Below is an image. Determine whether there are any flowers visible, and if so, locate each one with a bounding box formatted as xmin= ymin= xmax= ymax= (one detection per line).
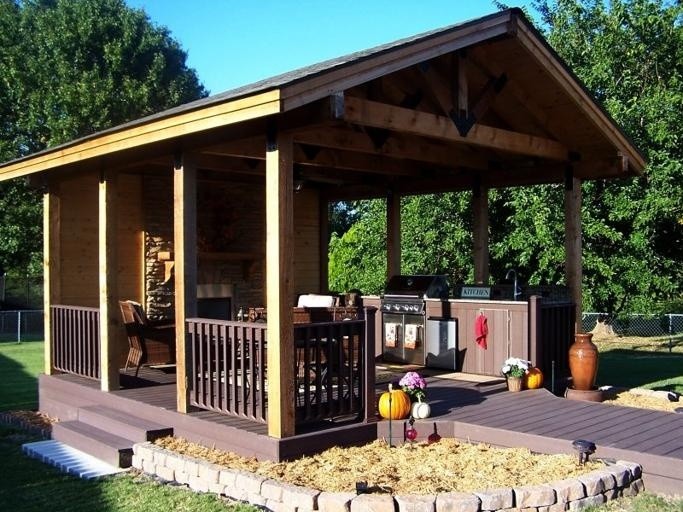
xmin=500 ymin=355 xmax=532 ymax=377
xmin=398 ymin=371 xmax=427 ymax=400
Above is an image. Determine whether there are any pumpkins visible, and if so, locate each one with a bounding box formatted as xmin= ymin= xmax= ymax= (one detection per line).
xmin=411 ymin=394 xmax=431 ymax=419
xmin=378 ymin=383 xmax=411 ymax=420
xmin=524 ymin=364 xmax=543 ymax=389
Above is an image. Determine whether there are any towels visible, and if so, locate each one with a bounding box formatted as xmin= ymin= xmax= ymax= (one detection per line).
xmin=384 ymin=322 xmax=418 ymax=350
xmin=473 ymin=313 xmax=489 ymax=351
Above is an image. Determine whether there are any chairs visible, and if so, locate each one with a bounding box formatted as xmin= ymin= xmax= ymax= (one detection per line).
xmin=116 ymin=298 xmax=211 ymax=379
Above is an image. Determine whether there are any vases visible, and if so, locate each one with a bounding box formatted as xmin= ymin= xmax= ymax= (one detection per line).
xmin=505 ymin=374 xmax=522 ymax=393
xmin=568 ymin=333 xmax=599 ymax=390
